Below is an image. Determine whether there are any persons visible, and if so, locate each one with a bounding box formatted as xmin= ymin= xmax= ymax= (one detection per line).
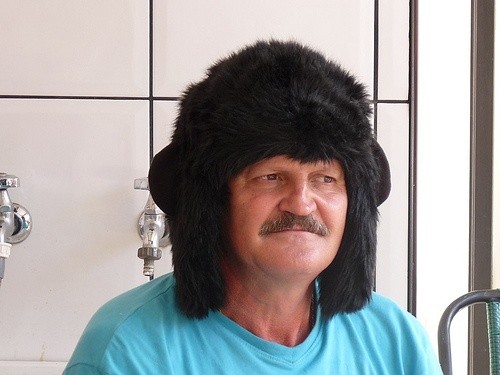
xmin=61 ymin=38 xmax=446 ymax=375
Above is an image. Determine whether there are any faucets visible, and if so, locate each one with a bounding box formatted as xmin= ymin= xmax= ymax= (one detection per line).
xmin=134 ymin=178 xmax=170 ymax=282
xmin=0 ymin=172 xmax=32 ymax=285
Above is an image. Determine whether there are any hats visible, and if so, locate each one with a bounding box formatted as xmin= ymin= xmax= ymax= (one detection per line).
xmin=147 ymin=37 xmax=391 ymax=321
